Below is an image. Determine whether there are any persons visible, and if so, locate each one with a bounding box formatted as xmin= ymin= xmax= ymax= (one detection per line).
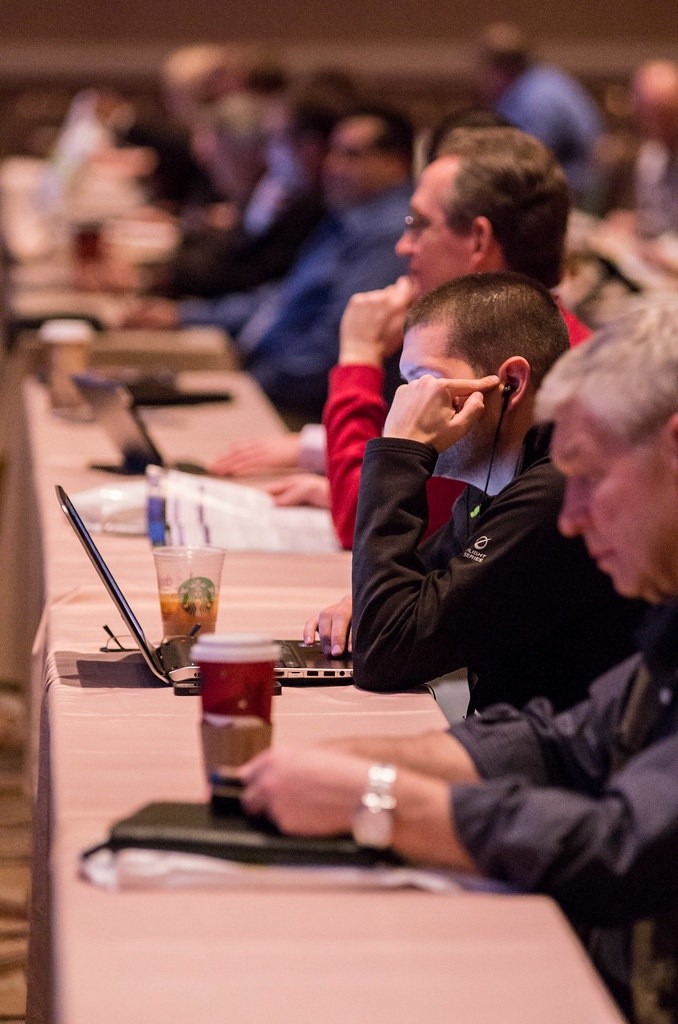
xmin=99 ymin=20 xmax=678 ymax=1024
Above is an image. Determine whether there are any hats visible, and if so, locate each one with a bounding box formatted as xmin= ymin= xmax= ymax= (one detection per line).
xmin=496 ymin=69 xmax=608 ymax=189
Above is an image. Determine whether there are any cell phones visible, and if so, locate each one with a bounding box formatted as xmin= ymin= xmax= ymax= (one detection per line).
xmin=209 ymin=774 xmax=245 ymax=797
xmin=174 ymin=682 xmax=282 ymax=696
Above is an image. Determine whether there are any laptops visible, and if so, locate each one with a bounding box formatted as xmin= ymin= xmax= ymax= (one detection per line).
xmin=107 ymin=801 xmax=406 ymax=870
xmin=55 ymin=483 xmax=358 ymax=683
xmin=70 ymin=375 xmax=221 ymax=479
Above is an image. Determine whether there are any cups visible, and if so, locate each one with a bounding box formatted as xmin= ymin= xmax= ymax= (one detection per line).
xmin=153 ymin=545 xmax=226 ymax=637
xmin=190 ymin=632 xmax=283 ymax=798
xmin=36 ymin=318 xmax=95 ymax=418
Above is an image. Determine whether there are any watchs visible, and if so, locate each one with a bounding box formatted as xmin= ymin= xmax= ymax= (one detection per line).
xmin=350 ymin=762 xmax=399 ymax=849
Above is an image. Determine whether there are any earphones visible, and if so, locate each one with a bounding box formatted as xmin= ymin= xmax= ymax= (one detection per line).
xmin=501 ymin=385 xmax=517 ymax=409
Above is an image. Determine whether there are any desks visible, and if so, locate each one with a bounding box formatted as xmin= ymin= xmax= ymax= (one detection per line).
xmin=0 ymin=370 xmax=631 ymax=1024
xmin=0 ymin=152 xmax=241 ymax=371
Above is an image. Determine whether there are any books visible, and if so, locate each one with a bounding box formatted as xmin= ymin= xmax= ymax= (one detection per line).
xmin=146 ymin=464 xmax=343 ymax=553
xmin=109 ymin=802 xmax=377 ymax=865
